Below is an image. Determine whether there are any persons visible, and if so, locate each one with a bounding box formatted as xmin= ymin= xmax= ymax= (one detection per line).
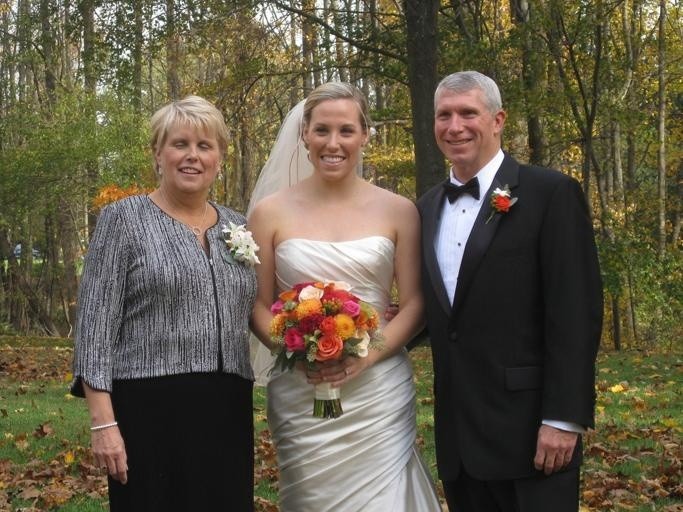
xmin=246 ymin=83 xmax=440 ymax=512
xmin=70 ymin=95 xmax=399 ymax=512
xmin=402 ymin=70 xmax=603 ymax=512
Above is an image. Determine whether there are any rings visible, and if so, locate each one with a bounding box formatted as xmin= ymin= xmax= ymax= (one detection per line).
xmin=100 ymin=465 xmax=106 ymax=470
xmin=344 ymin=369 xmax=348 ymax=375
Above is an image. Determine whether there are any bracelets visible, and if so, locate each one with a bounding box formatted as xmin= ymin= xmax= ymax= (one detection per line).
xmin=88 ymin=421 xmax=120 ymax=431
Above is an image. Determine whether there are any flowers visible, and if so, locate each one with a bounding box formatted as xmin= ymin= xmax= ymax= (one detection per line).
xmin=269 ymin=282 xmax=386 ymax=419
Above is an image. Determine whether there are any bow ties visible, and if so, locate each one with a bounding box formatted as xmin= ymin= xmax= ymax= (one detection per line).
xmin=443 ymin=177 xmax=482 ymax=205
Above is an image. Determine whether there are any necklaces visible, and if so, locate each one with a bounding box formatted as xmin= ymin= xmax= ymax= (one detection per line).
xmin=159 ymin=192 xmax=207 ymax=237
